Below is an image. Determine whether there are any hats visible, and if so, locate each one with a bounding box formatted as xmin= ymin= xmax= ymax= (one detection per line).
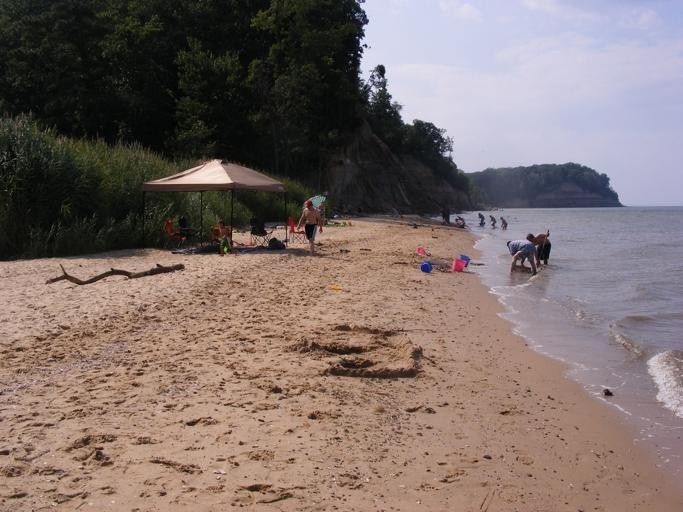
xmin=305 ymin=200 xmax=313 ymax=207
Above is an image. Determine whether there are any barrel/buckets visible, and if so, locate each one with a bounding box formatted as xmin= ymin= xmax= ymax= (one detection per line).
xmin=453 ymin=257 xmax=466 ymax=271
xmin=421 ymin=261 xmax=432 ymax=272
xmin=461 ymin=254 xmax=472 ymax=267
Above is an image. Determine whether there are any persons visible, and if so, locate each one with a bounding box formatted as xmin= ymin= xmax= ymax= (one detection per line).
xmin=525 ymin=229 xmax=550 ymax=266
xmin=438 ymin=207 xmax=468 ymax=229
xmin=489 ymin=215 xmax=496 ymax=229
xmin=500 ymin=217 xmax=506 ymax=230
xmin=296 ymin=200 xmax=323 ymax=256
xmin=287 ymin=213 xmax=294 ymax=234
xmin=506 ymin=240 xmax=537 ymax=276
xmin=212 ymin=221 xmax=229 ymax=251
xmin=163 ymin=215 xmax=186 ymax=242
xmin=477 ymin=213 xmax=485 ymax=226
xmin=316 ymin=202 xmax=324 ymax=227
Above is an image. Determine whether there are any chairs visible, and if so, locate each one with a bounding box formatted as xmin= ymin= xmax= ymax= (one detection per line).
xmin=288 ymin=216 xmax=307 ymax=244
xmin=177 ymin=212 xmax=204 ymax=251
xmin=250 ymin=217 xmax=272 ymax=247
